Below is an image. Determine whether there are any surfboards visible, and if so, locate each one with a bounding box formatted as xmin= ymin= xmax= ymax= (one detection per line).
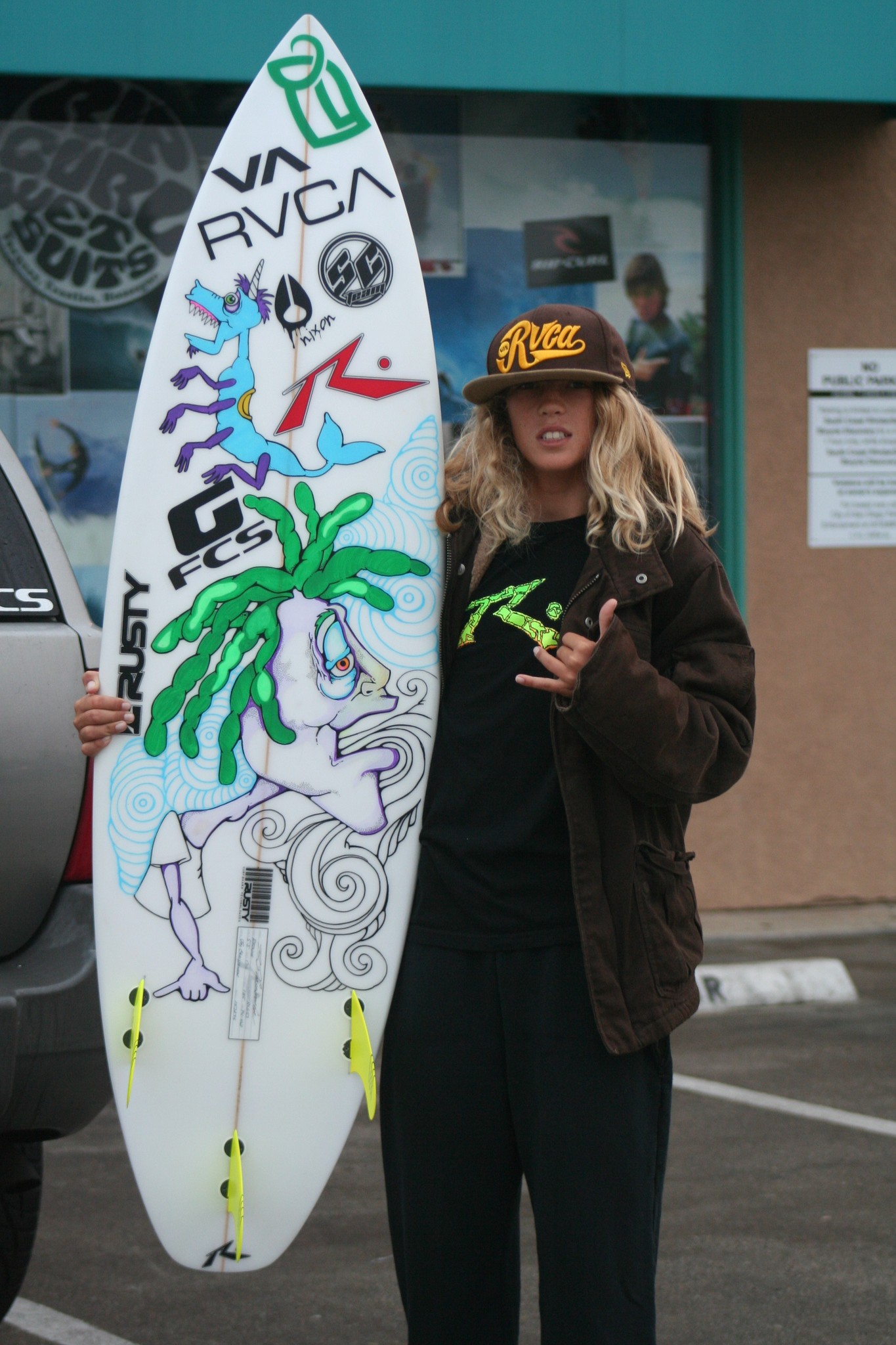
xmin=92 ymin=14 xmax=444 ymax=1275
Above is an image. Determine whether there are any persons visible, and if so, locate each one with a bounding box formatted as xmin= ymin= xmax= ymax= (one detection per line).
xmin=621 ymin=250 xmax=693 ymax=415
xmin=41 ymin=419 xmax=87 ymax=498
xmin=75 ymin=304 xmax=755 ymax=1345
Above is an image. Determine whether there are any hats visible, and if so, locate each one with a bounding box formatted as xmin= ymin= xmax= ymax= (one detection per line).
xmin=461 ymin=304 xmax=639 ymax=406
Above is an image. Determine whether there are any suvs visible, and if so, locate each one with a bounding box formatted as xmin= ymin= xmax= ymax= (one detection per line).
xmin=1 ymin=427 xmax=115 ymax=1320
